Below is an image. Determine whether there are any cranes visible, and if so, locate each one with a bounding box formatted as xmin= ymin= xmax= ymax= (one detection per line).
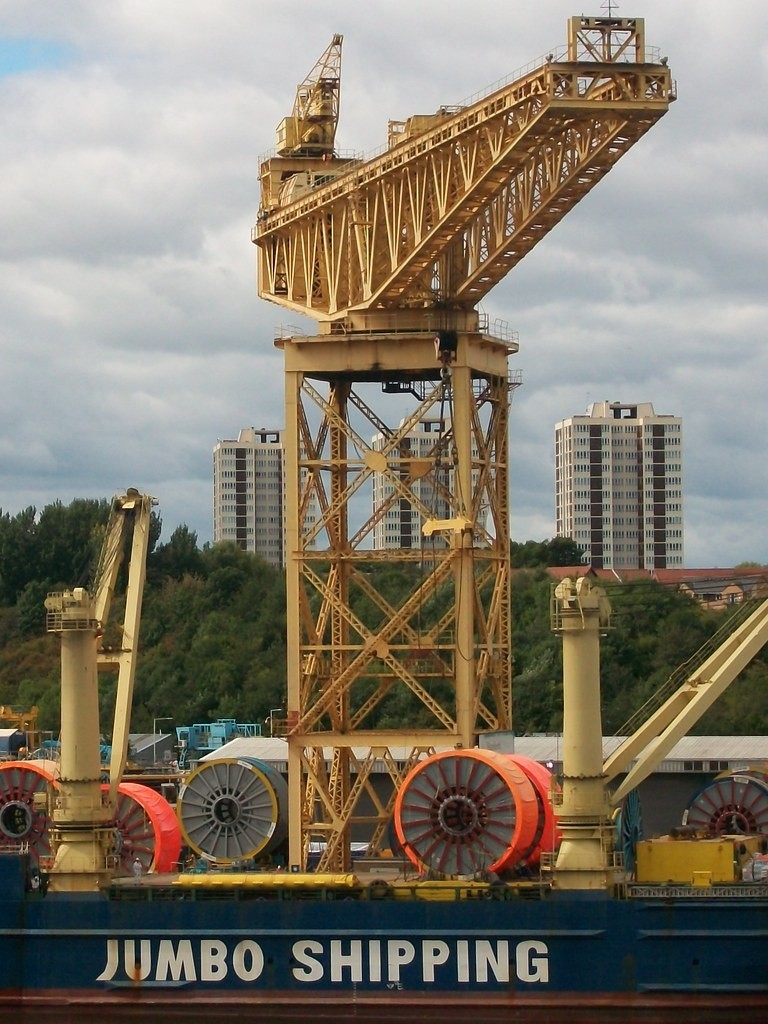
xmin=249 ymin=1 xmax=677 ymax=900
